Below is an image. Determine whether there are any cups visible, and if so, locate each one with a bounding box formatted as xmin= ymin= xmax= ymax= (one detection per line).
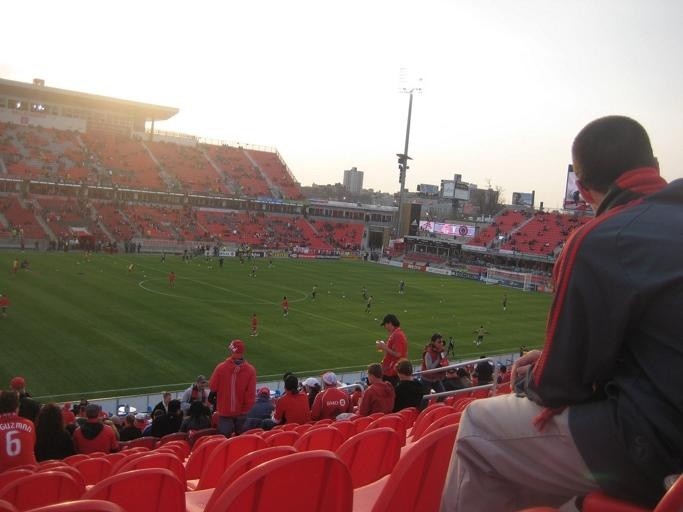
xmin=440 ymin=352 xmax=445 ymax=359
xmin=375 ymin=341 xmax=383 ymax=352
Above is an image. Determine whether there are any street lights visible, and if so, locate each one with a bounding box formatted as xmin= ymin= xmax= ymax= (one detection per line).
xmin=392 ymin=81 xmax=422 ymax=246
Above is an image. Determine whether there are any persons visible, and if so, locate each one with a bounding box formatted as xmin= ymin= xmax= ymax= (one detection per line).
xmin=3 ymin=117 xmax=682 ymax=509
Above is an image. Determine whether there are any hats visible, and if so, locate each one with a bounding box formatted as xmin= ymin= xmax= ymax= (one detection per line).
xmin=302 ymin=373 xmax=337 ymax=388
xmin=134 ymin=413 xmax=144 ymax=419
xmin=230 ymin=339 xmax=244 ymax=354
xmin=197 ymin=376 xmax=208 ymax=383
xmin=11 ymin=377 xmax=24 ymax=388
xmin=258 ymin=388 xmax=270 ymax=395
xmin=380 ymin=315 xmax=396 ymax=326
